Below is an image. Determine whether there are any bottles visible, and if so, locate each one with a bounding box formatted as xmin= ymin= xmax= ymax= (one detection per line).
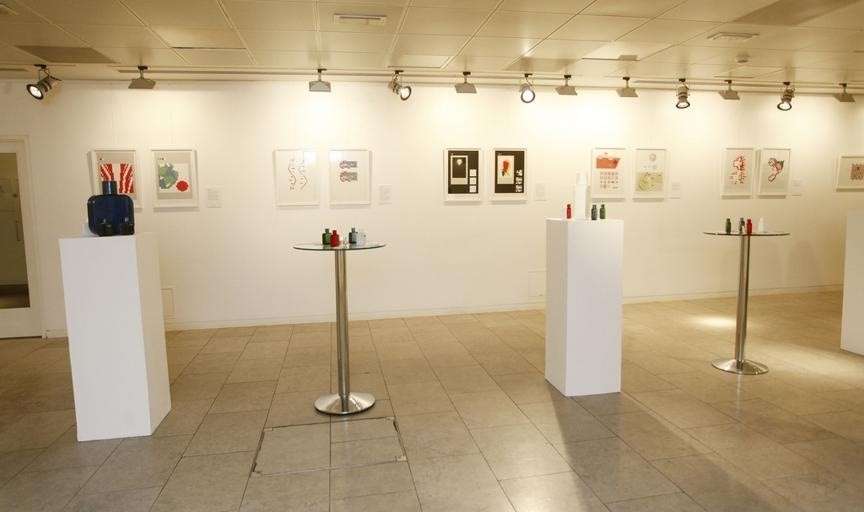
xmin=565 ymin=199 xmax=609 ymax=222
xmin=319 ymin=224 xmax=368 ymax=246
xmin=715 ymin=211 xmax=770 ymax=236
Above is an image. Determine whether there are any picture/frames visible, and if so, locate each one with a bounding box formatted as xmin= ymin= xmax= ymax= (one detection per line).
xmin=327 ymin=148 xmax=371 ymax=207
xmin=89 ymin=148 xmax=144 ymax=210
xmin=150 ymin=147 xmax=199 ymax=209
xmin=443 ymin=147 xmax=483 ymax=203
xmin=589 ymin=145 xmax=627 ymax=199
xmin=720 ymin=147 xmax=756 ymax=196
xmin=836 ymin=155 xmax=864 ymax=190
xmin=273 ymin=148 xmax=320 ymax=206
xmin=757 ymin=147 xmax=792 ymax=196
xmin=488 ymin=148 xmax=527 ymax=202
xmin=631 ymin=146 xmax=667 ymax=199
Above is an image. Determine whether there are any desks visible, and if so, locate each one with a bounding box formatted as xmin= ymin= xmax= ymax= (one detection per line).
xmin=293 ymin=242 xmax=387 ymax=417
xmin=704 ymin=231 xmax=790 ymax=375
